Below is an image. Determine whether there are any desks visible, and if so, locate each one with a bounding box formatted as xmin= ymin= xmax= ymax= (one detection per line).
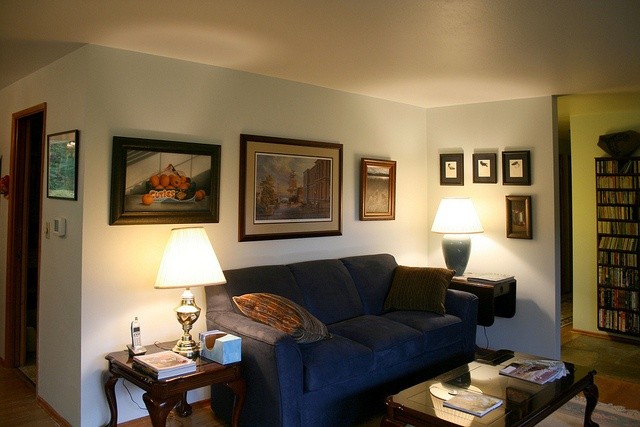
xmin=104 ymin=340 xmax=246 ymax=427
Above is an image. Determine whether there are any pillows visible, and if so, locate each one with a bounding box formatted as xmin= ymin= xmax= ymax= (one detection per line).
xmin=232 ymin=292 xmax=334 ymax=345
xmin=382 ymin=265 xmax=457 ymax=317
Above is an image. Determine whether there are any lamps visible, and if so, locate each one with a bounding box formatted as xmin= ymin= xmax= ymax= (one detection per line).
xmin=431 ymin=198 xmax=485 ymax=276
xmin=153 ymin=227 xmax=228 ymax=359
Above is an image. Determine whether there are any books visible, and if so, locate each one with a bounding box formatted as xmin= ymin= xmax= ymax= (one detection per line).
xmin=442 ymin=391 xmax=504 ymax=416
xmin=596 ymin=160 xmax=639 ymax=174
xmin=598 ymin=250 xmax=638 ymax=268
xmin=597 ymin=191 xmax=639 ymax=205
xmin=598 ymin=287 xmax=639 ymax=311
xmin=467 ymin=272 xmax=515 ymax=285
xmin=497 ymin=357 xmax=569 ymax=386
xmin=596 ymin=176 xmax=640 ymax=190
xmin=132 ymin=350 xmax=199 ymax=380
xmin=598 ymin=207 xmax=633 ymax=220
xmin=598 ymin=222 xmax=638 ymax=236
xmin=598 ymin=266 xmax=639 ymax=290
xmin=598 ymin=237 xmax=638 ymax=252
xmin=599 ymin=308 xmax=640 ymax=338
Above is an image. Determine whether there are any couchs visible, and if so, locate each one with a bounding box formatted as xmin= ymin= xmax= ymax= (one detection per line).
xmin=205 ymin=253 xmax=479 ymax=427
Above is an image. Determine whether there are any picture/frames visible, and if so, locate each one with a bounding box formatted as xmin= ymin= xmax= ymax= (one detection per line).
xmin=238 ymin=133 xmax=343 ymax=242
xmin=47 ymin=129 xmax=80 ymax=201
xmin=472 ymin=153 xmax=497 ymax=183
xmin=359 ymin=157 xmax=398 ymax=221
xmin=502 ymin=150 xmax=531 ymax=186
xmin=440 ymin=153 xmax=464 ymax=186
xmin=109 ymin=135 xmax=222 ymax=226
xmin=505 ymin=195 xmax=533 ymax=240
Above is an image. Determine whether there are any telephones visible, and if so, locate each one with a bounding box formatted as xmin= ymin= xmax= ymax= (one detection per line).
xmin=126 ymin=317 xmax=148 ymax=358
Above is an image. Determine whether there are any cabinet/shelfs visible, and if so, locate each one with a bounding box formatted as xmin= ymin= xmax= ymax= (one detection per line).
xmin=595 ymin=158 xmax=640 ymax=339
